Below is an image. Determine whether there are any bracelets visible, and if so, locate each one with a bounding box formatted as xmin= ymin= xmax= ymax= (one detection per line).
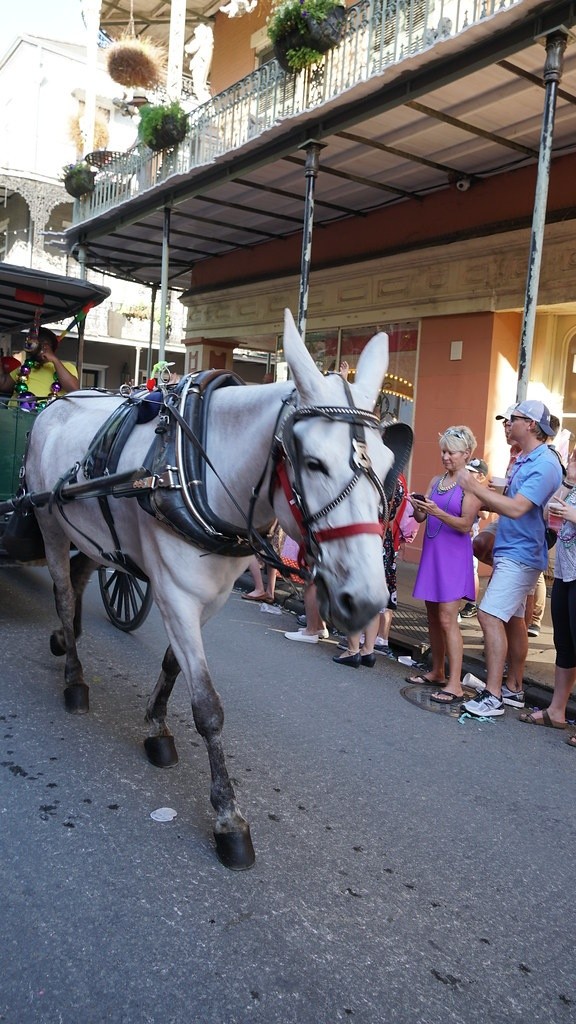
xmin=562 ymin=480 xmax=573 ymax=489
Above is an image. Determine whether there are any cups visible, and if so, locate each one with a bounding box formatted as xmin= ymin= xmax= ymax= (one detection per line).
xmin=463 ymin=673 xmax=486 ymax=691
xmin=547 ymin=502 xmax=563 ymax=530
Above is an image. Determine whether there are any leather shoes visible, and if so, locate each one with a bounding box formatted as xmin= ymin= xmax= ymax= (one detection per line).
xmin=360 ymin=652 xmax=376 ymax=667
xmin=333 ymin=653 xmax=361 ymax=670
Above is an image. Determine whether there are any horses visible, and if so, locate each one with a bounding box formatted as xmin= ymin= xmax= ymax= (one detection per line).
xmin=25 ymin=308 xmax=393 ymax=871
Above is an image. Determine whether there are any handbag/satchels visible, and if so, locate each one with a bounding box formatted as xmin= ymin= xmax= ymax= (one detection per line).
xmin=545 ymin=529 xmax=558 ymax=550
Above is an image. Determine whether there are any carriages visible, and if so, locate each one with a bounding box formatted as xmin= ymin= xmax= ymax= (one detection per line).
xmin=0 ymin=263 xmax=390 ymax=870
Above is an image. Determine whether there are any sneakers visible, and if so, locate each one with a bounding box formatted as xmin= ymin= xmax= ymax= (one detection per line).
xmin=527 ymin=625 xmax=540 ymax=636
xmin=476 ymin=683 xmax=526 ymax=708
xmin=460 ymin=603 xmax=477 ymax=618
xmin=461 ymin=690 xmax=505 ymax=717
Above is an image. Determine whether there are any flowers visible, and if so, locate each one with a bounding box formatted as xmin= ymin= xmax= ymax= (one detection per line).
xmin=268 ymin=0 xmax=348 ymax=47
xmin=57 ymin=163 xmax=91 ymax=186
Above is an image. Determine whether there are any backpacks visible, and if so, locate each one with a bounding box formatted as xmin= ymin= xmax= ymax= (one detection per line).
xmin=390 ymin=475 xmax=420 ymax=551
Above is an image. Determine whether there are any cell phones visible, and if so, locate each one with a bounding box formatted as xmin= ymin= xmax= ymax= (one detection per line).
xmin=411 ymin=494 xmax=427 ymax=504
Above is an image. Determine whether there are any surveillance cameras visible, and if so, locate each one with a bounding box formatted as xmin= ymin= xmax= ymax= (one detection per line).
xmin=457 ymin=178 xmax=470 ymax=192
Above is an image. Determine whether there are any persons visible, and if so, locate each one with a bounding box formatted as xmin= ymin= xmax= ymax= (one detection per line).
xmin=185 ymin=24 xmax=214 ymax=104
xmin=241 ymin=556 xmax=277 ymax=604
xmin=128 ymin=90 xmax=153 ymax=191
xmin=284 ymin=473 xmax=408 ymax=669
xmin=520 ymin=445 xmax=576 ymax=748
xmin=404 ymin=425 xmax=482 ymax=703
xmin=0 ymin=327 xmax=79 ymax=410
xmin=458 ymin=399 xmax=564 ymax=717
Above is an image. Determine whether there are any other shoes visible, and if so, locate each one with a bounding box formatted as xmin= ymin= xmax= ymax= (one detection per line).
xmin=240 ymin=591 xmax=266 ymax=602
xmin=338 ymin=638 xmax=390 ymax=655
xmin=297 ymin=615 xmax=307 ymax=625
xmin=284 ymin=631 xmax=319 ymax=643
xmin=263 ymin=596 xmax=275 ymax=604
xmin=298 ymin=627 xmax=330 ymax=639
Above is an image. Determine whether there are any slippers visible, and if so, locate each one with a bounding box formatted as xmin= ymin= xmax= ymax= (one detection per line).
xmin=431 ymin=689 xmax=464 ymax=703
xmin=519 ymin=708 xmax=567 ymax=729
xmin=567 ymin=734 xmax=576 ymax=746
xmin=405 ymin=674 xmax=446 ymax=687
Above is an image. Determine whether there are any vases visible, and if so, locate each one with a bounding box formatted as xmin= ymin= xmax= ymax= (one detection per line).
xmin=64 ymin=171 xmax=95 ymax=198
xmin=271 ymin=6 xmax=344 ymax=73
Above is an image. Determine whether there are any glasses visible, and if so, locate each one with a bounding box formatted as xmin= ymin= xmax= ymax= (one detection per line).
xmin=502 ymin=422 xmax=506 ymax=427
xmin=510 ymin=414 xmax=531 ymax=422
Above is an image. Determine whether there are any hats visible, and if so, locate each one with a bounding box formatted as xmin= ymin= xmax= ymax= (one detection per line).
xmin=495 ymin=403 xmax=518 ymax=421
xmin=515 ymin=400 xmax=556 ymax=435
xmin=464 ymin=459 xmax=488 ymax=476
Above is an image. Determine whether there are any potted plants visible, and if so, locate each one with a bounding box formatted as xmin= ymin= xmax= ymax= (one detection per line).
xmin=137 ymin=99 xmax=189 ymax=152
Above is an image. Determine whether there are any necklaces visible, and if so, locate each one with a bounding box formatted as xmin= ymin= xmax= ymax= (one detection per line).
xmin=16 ymin=361 xmax=62 ymax=408
xmin=426 ymin=472 xmax=457 ymax=538
